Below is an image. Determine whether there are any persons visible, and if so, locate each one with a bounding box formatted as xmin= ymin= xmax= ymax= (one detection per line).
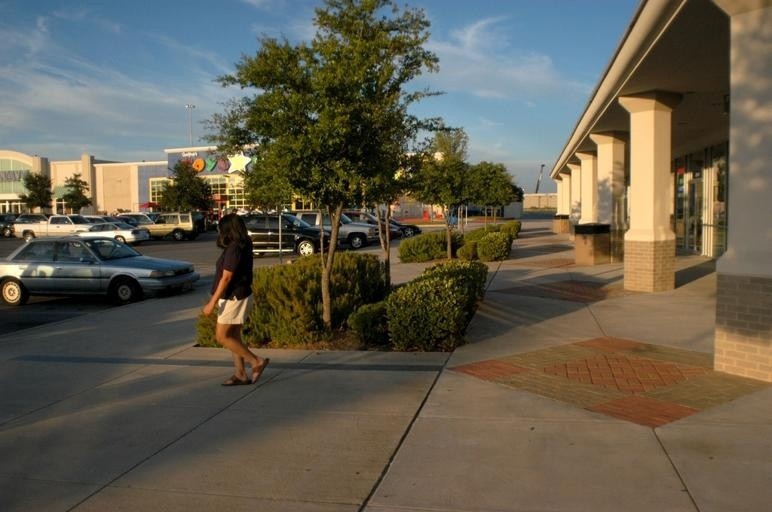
xmin=202 ymin=213 xmax=269 ymax=386
xmin=232 ymin=208 xmax=238 ymax=214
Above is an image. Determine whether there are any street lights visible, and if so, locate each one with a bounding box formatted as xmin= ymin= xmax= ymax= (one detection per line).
xmin=184 ymin=104 xmax=196 ymax=146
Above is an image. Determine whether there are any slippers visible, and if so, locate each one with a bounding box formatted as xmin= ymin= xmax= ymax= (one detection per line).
xmin=221 ymin=374 xmax=251 ymax=386
xmin=252 ymin=358 xmax=269 ymax=383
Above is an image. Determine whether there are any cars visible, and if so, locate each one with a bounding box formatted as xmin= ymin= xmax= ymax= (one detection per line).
xmin=241 ymin=210 xmax=422 ymax=256
xmin=0 ymin=211 xmax=207 ymax=305
